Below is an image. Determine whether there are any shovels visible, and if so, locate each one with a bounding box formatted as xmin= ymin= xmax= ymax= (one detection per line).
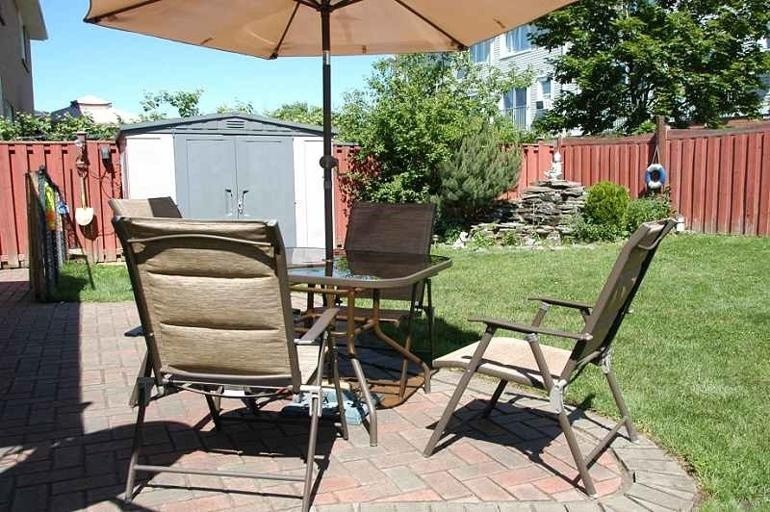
xmin=75 ymin=167 xmax=94 ymax=227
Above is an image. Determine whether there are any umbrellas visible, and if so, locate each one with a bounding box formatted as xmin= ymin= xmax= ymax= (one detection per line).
xmin=82 ymin=0 xmax=576 ymax=426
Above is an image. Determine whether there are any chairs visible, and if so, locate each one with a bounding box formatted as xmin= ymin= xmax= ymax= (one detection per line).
xmin=422 ymin=218 xmax=676 ymax=500
xmin=312 ymin=204 xmax=436 ymax=368
xmin=108 ymin=196 xmax=182 ymax=218
xmin=111 ymin=217 xmax=348 ymax=512
